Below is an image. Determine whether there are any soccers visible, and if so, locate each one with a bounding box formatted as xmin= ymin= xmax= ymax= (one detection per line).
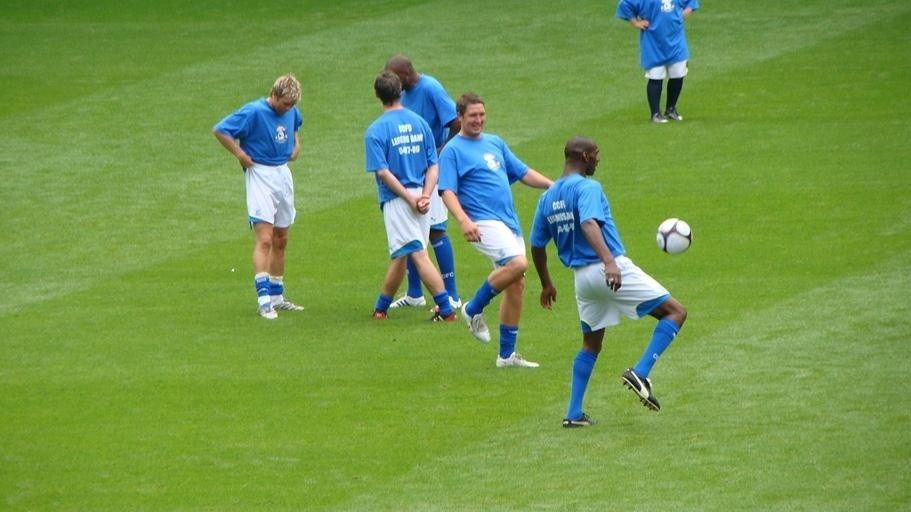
xmin=656 ymin=218 xmax=691 ymax=254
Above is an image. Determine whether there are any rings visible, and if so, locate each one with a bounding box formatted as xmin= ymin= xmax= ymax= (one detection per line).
xmin=608 ymin=278 xmax=614 ymax=283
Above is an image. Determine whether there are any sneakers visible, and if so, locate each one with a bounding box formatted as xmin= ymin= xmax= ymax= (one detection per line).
xmin=259 ymin=302 xmax=278 ymax=319
xmin=651 ymin=112 xmax=668 ymax=123
xmin=373 ymin=310 xmax=389 ymax=320
xmin=496 ymin=352 xmax=539 ymax=368
xmin=273 ymin=300 xmax=304 ymax=310
xmin=665 ymin=107 xmax=683 ymax=120
xmin=563 ymin=413 xmax=597 ymax=428
xmin=623 ymin=367 xmax=660 ymax=412
xmin=390 ymin=293 xmax=426 ymax=308
xmin=431 ymin=296 xmax=461 ymax=322
xmin=461 ymin=301 xmax=491 ymax=343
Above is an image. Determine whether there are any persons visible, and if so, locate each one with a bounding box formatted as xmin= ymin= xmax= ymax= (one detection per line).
xmin=614 ymin=0 xmax=700 ymax=123
xmin=383 ymin=52 xmax=462 ymax=311
xmin=212 ymin=71 xmax=306 ymax=320
xmin=529 ymin=132 xmax=686 ymax=429
xmin=436 ymin=89 xmax=556 ymax=367
xmin=364 ymin=68 xmax=458 ymax=322
